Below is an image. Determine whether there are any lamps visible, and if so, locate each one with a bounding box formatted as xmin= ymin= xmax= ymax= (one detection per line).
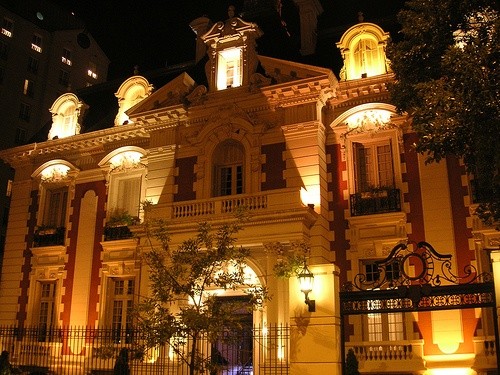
xmin=296 ymin=263 xmax=315 ymax=312
